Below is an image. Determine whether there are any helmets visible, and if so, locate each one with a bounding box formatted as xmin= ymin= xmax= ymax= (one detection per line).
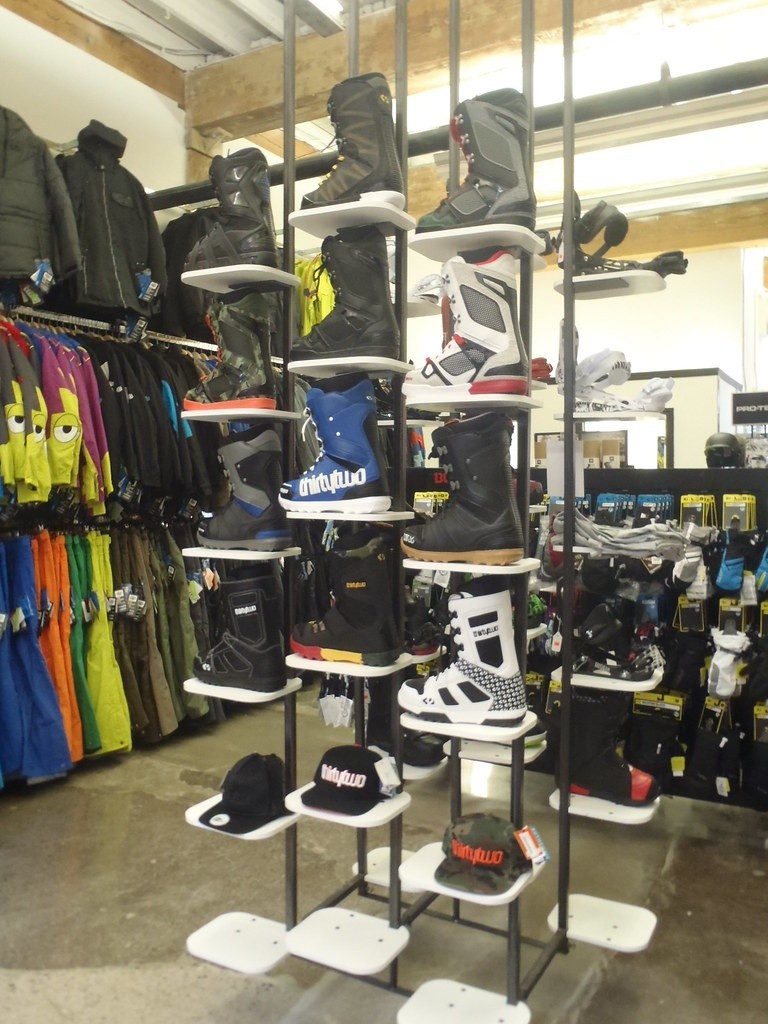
xmin=704 ymin=432 xmax=741 ymax=462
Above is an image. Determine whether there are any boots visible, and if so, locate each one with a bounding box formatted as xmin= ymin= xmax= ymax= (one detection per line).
xmin=299 ymin=72 xmax=405 ymax=211
xmin=288 ymin=226 xmax=400 ymax=359
xmin=398 ymin=575 xmax=528 ymax=725
xmin=181 ymin=147 xmax=277 ymax=272
xmin=416 ymin=88 xmax=536 ymax=233
xmin=276 ymin=371 xmax=393 ymax=514
xmin=180 ymin=286 xmax=276 ymax=410
xmin=288 ymin=526 xmax=401 ymax=666
xmin=367 ymin=663 xmax=446 ymax=766
xmin=553 ymin=684 xmax=661 ymax=805
xmin=399 ymin=246 xmax=528 ymax=395
xmin=399 ymin=410 xmax=525 ymax=565
xmin=197 ymin=424 xmax=293 ymax=550
xmin=194 ymin=558 xmax=287 ymax=692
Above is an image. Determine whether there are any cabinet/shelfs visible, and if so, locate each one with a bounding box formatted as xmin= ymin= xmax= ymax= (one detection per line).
xmin=181 ymin=0 xmax=669 ymax=1024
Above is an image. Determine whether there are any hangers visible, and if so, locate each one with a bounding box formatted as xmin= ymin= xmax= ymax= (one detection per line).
xmin=0 ymin=514 xmax=191 ymax=540
xmin=0 ymin=304 xmax=284 ymax=378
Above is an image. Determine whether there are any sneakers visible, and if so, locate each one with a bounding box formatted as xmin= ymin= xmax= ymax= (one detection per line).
xmin=402 ymin=594 xmax=440 ymax=655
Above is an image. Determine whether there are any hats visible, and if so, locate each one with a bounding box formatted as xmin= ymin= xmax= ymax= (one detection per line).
xmin=435 ymin=813 xmax=532 ymax=896
xmin=301 ymin=743 xmax=402 ymax=817
xmin=198 ymin=752 xmax=294 ymax=834
